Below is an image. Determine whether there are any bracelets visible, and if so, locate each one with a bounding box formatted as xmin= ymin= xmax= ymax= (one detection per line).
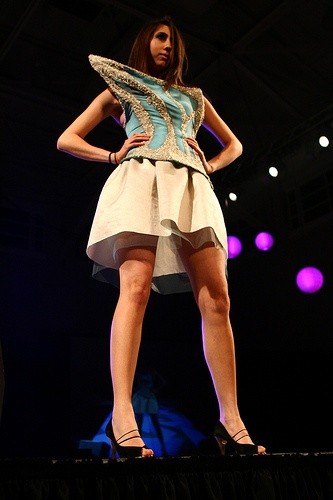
xmin=114 ymin=151 xmax=119 ymax=165
xmin=109 ymin=152 xmax=113 ymax=164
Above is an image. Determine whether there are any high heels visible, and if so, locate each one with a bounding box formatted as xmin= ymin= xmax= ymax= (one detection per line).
xmin=214 ymin=419 xmax=265 ymax=455
xmin=104 ymin=417 xmax=154 ymax=459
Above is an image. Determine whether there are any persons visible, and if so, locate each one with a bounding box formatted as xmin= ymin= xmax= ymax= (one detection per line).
xmin=56 ymin=14 xmax=271 ymax=456
